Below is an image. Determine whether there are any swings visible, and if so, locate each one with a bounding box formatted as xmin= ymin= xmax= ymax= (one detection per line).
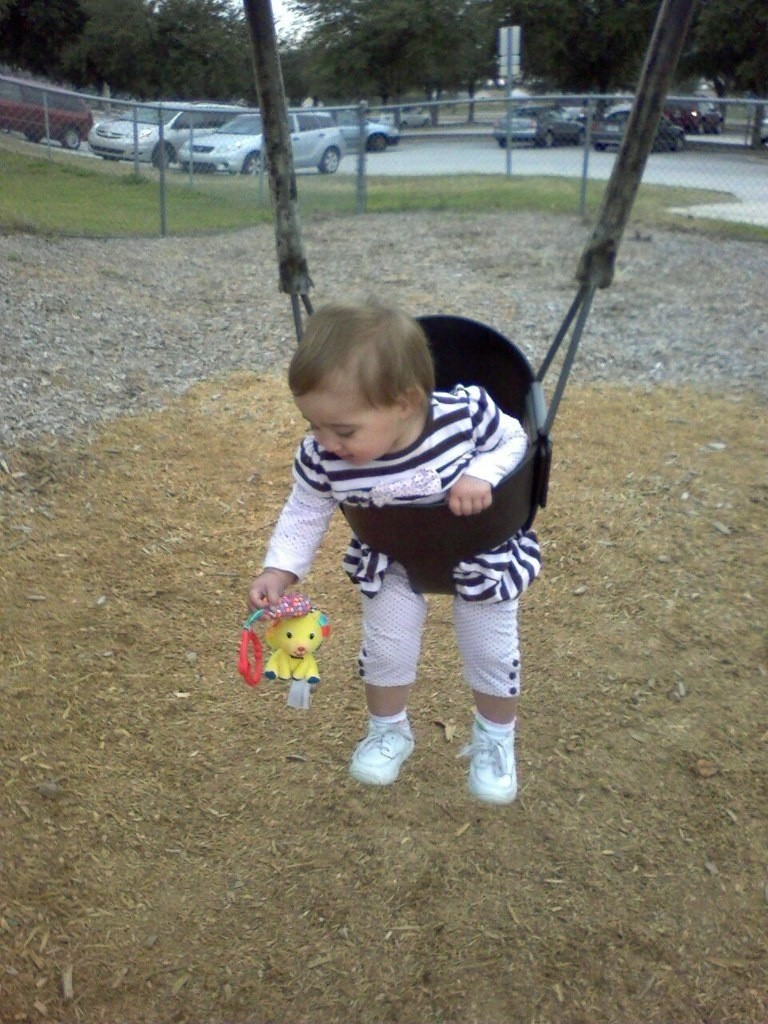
xmin=241 ymin=1 xmax=698 ymax=596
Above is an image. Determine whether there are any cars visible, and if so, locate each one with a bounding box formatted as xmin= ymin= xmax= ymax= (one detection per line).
xmin=493 ymin=105 xmax=587 ymax=149
xmin=662 ymin=95 xmax=725 ymax=135
xmin=586 ymin=102 xmax=687 ymax=154
xmin=380 ymin=106 xmax=431 ymax=128
xmin=176 ymin=109 xmax=344 ymax=177
xmin=333 ymin=108 xmax=402 ymax=155
xmin=0 ymin=79 xmax=94 ymax=150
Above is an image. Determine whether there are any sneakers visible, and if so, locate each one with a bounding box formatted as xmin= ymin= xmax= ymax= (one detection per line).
xmin=349 ymin=719 xmax=414 ymax=786
xmin=457 ymin=724 xmax=517 ymax=804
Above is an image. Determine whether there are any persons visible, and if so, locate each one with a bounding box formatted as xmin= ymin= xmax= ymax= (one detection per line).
xmin=248 ymin=303 xmax=542 ymax=806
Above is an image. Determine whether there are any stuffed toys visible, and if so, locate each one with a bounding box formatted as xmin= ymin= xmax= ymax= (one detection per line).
xmin=264 ymin=607 xmax=330 ymax=684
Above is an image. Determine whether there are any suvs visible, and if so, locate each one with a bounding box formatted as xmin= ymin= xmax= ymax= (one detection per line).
xmin=86 ymin=99 xmax=250 ymax=169
